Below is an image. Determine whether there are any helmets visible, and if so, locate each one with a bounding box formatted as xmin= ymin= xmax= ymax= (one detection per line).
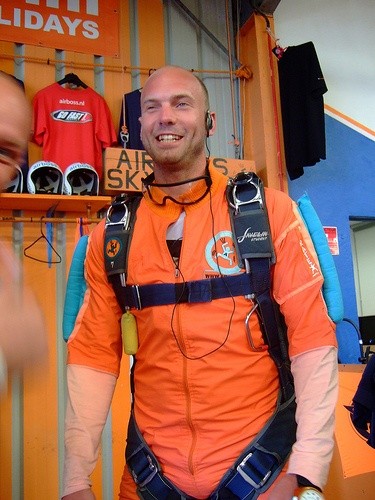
xmin=1 ymin=161 xmax=23 ymax=193
xmin=64 ymin=162 xmax=99 ymax=196
xmin=26 ymin=161 xmax=64 ymax=195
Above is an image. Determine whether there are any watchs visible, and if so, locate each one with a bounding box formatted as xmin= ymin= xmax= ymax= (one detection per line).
xmin=290 ymin=486 xmax=326 ymax=500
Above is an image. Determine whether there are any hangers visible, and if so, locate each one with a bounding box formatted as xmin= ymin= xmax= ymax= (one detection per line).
xmin=139 ymin=68 xmax=156 ymax=91
xmin=24 ymin=216 xmax=62 ymax=264
xmin=56 ymin=62 xmax=88 ymax=89
xmin=275 ymin=39 xmax=287 ymax=59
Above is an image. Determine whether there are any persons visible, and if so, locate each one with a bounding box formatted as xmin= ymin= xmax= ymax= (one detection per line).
xmin=0 ymin=69 xmax=48 ymax=377
xmin=65 ymin=67 xmax=338 ymax=500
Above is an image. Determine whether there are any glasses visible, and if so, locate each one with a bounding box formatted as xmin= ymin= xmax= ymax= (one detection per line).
xmin=141 ymin=157 xmax=212 ymax=207
